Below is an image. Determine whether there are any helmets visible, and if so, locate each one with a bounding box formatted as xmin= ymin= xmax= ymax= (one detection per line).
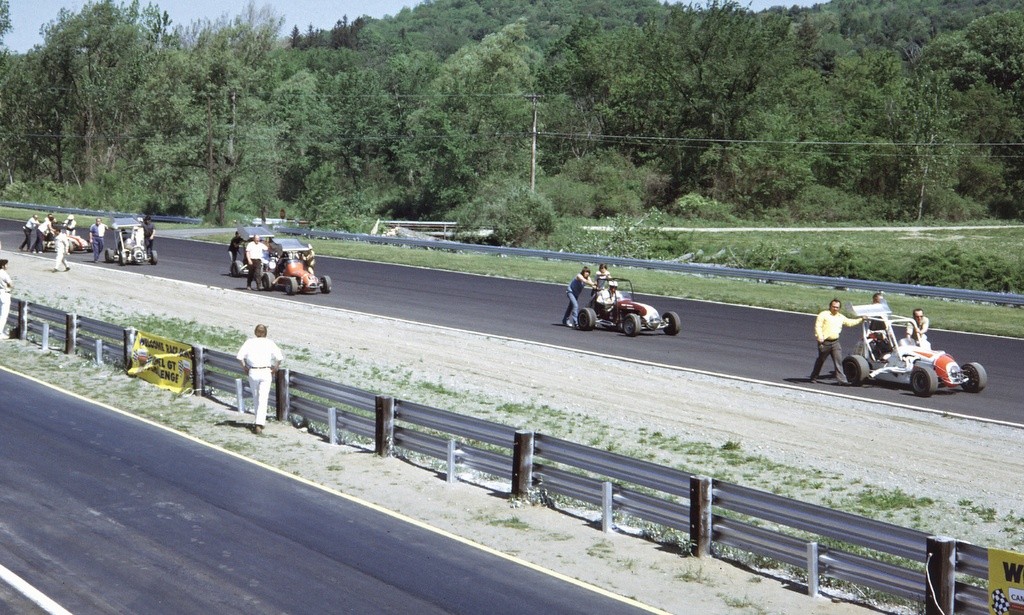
xmin=608 ymin=281 xmax=618 ymax=286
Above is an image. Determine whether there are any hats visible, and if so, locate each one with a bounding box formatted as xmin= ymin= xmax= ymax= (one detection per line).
xmin=68 ymin=214 xmax=74 ymax=220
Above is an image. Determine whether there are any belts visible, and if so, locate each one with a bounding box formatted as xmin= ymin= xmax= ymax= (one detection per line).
xmin=250 ymin=367 xmax=271 ymax=369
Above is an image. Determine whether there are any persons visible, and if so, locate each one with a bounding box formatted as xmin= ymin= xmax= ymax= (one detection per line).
xmin=30 ymin=217 xmax=56 ymax=253
xmin=810 ymin=299 xmax=865 ymax=384
xmin=904 ymin=308 xmax=929 ymax=343
xmin=0 ymin=259 xmax=15 ymax=335
xmin=867 ymin=291 xmax=887 ymax=330
xmin=235 ymin=324 xmax=260 ymax=435
xmin=18 ymin=215 xmax=41 ymax=251
xmin=89 ymin=218 xmax=109 ymax=263
xmin=142 ymin=216 xmax=156 ymax=257
xmin=223 ymin=221 xmax=318 ymax=293
xmin=591 ymin=264 xmax=612 ymax=297
xmin=44 ymin=213 xmax=58 ymax=224
xmin=62 ymin=215 xmax=77 ymax=237
xmin=52 ymin=228 xmax=71 ymax=273
xmin=600 ymin=280 xmax=623 ymax=312
xmin=561 ymin=265 xmax=597 ymax=329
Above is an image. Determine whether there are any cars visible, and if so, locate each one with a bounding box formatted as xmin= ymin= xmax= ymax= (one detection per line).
xmin=105 ymin=214 xmax=158 ymax=265
xmin=576 ymin=272 xmax=681 ymax=338
xmin=40 ymin=219 xmax=94 ymax=253
xmin=261 ymin=237 xmax=332 ymax=294
xmin=229 ymin=225 xmax=277 ymax=278
xmin=841 ymin=302 xmax=988 ymax=397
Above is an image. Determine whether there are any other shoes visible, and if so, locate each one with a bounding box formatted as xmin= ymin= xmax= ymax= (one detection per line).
xmin=247 ymin=286 xmax=252 ymax=290
xmin=562 ymin=320 xmax=572 ymax=327
xmin=810 ymin=378 xmax=817 ymax=383
xmin=255 ymin=425 xmax=262 ymax=434
xmin=0 ymin=333 xmax=10 ymax=340
xmin=575 ymin=325 xmax=582 ymax=330
xmin=257 ymin=286 xmax=265 ymax=290
xmin=64 ymin=267 xmax=70 ymax=272
xmin=52 ymin=268 xmax=57 ymax=273
xmin=839 ymin=382 xmax=852 ymax=386
